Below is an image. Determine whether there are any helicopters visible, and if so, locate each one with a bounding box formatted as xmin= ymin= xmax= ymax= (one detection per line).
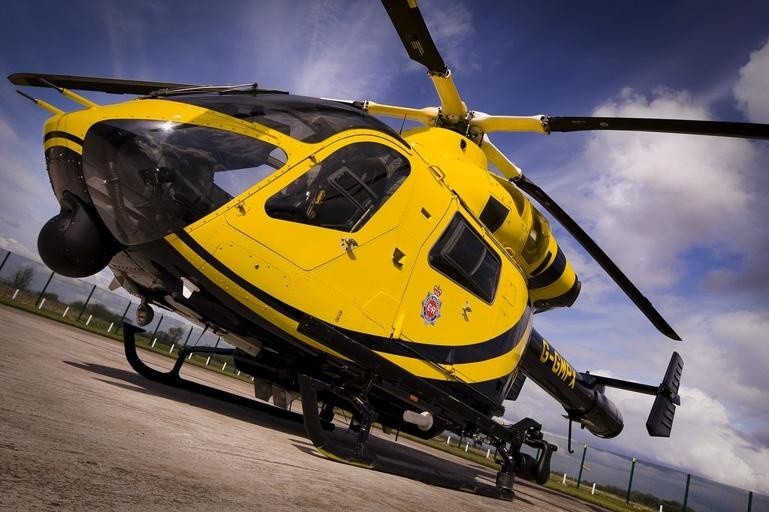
xmin=6 ymin=1 xmax=769 ymax=502
